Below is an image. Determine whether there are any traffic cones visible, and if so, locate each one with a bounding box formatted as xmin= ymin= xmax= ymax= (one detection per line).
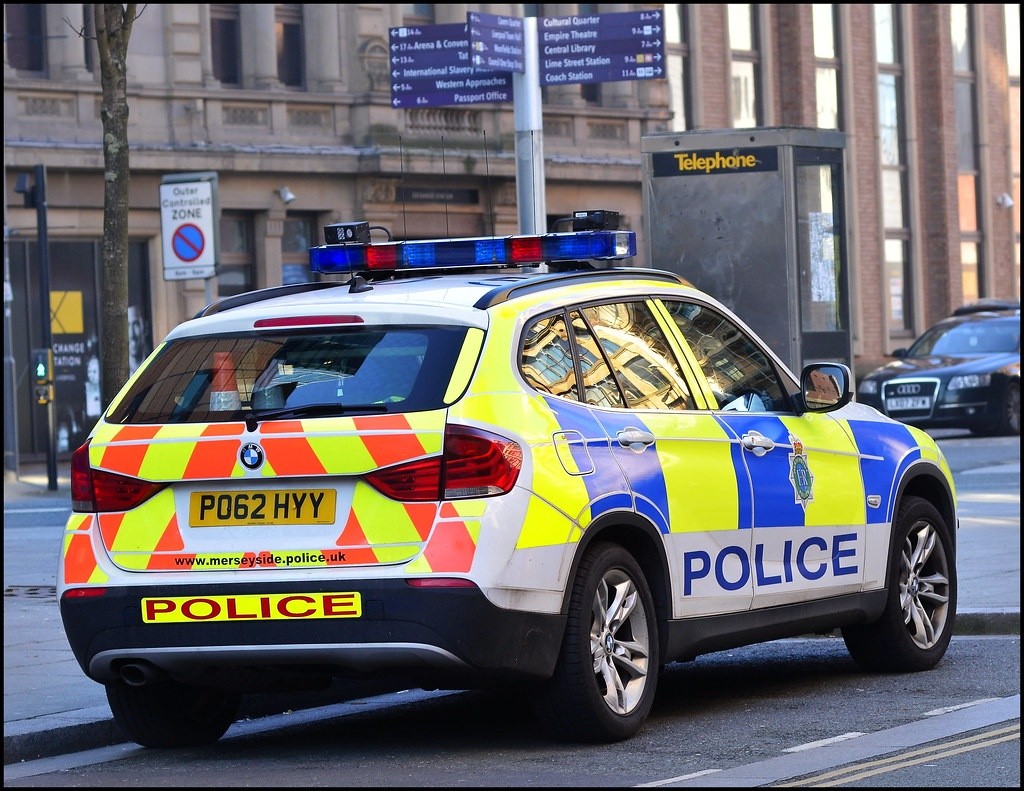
xmin=208 ymin=351 xmax=243 ymax=412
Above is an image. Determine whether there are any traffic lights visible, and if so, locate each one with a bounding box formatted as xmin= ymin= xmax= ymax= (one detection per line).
xmin=28 ymin=348 xmax=54 ymax=407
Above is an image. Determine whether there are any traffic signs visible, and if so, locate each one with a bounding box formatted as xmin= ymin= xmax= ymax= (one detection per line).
xmin=537 ymin=10 xmax=667 ymax=87
xmin=387 ymin=10 xmax=524 ymax=108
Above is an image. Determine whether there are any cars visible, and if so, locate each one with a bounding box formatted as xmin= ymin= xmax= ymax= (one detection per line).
xmin=855 ymin=300 xmax=1020 ymax=437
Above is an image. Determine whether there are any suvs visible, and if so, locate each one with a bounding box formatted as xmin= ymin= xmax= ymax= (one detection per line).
xmin=65 ymin=209 xmax=959 ymax=745
